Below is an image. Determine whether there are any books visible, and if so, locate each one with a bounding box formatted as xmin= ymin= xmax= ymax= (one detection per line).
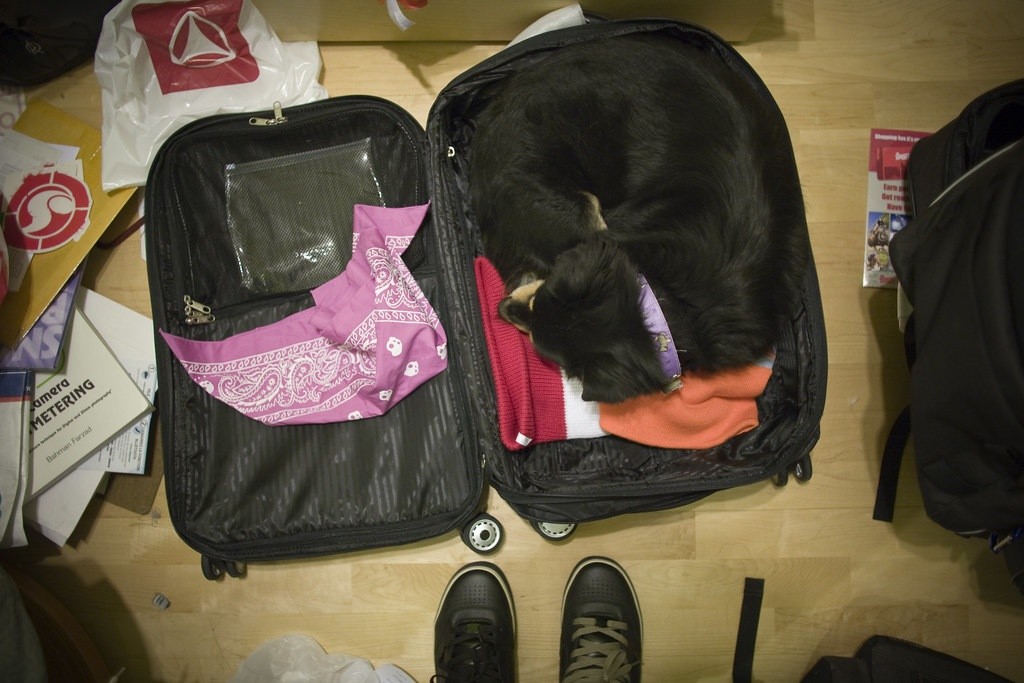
xmin=0 ymin=256 xmax=158 ymax=549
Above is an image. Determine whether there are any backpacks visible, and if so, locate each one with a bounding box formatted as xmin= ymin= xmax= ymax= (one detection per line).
xmin=871 ymin=78 xmax=1024 ymax=554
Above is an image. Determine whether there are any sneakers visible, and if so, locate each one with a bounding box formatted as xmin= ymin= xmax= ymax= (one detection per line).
xmin=559 ymin=555 xmax=643 ymax=683
xmin=430 ymin=561 xmax=516 ymax=683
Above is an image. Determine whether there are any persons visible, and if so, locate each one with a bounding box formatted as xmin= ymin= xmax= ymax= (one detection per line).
xmin=429 ymin=556 xmax=644 ymax=683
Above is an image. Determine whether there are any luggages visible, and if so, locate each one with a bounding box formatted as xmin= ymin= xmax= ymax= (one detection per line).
xmin=146 ymin=20 xmax=834 ymax=581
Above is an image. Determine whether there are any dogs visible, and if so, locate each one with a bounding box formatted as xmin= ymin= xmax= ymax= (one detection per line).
xmin=468 ymin=38 xmax=812 ymax=405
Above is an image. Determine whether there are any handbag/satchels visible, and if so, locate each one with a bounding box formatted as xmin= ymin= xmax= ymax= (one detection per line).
xmin=732 ymin=577 xmax=1016 ymax=683
xmin=95 ymin=0 xmax=329 ymax=192
